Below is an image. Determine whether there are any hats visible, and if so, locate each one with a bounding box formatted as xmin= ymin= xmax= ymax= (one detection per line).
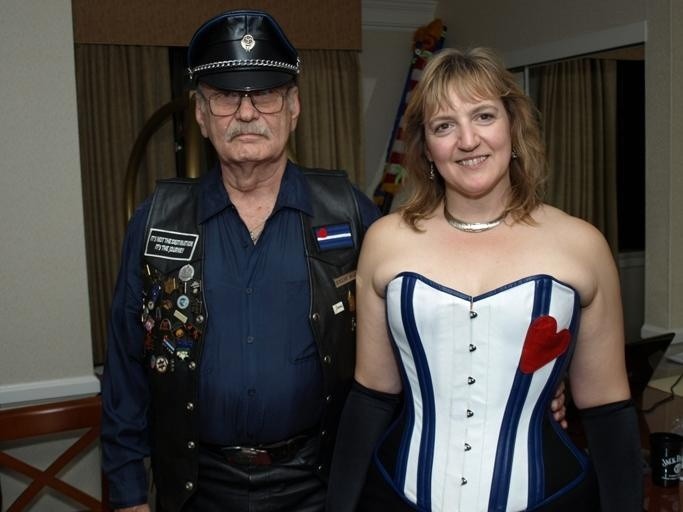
xmin=186 ymin=8 xmax=300 ymax=93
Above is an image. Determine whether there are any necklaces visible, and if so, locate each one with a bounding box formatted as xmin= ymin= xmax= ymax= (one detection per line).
xmin=439 ymin=197 xmax=509 ymax=231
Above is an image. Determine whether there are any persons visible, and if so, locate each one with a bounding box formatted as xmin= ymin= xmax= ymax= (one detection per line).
xmin=97 ymin=16 xmax=570 ymax=512
xmin=325 ymin=40 xmax=645 ymax=512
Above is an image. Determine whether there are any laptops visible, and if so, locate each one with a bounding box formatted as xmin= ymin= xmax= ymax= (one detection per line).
xmin=625 ymin=332 xmax=676 ymax=403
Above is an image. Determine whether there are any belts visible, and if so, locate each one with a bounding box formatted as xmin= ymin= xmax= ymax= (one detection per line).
xmin=201 ymin=438 xmax=313 ymax=471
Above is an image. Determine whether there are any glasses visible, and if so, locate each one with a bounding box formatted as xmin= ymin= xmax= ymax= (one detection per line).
xmin=194 ymin=81 xmax=289 ymax=118
